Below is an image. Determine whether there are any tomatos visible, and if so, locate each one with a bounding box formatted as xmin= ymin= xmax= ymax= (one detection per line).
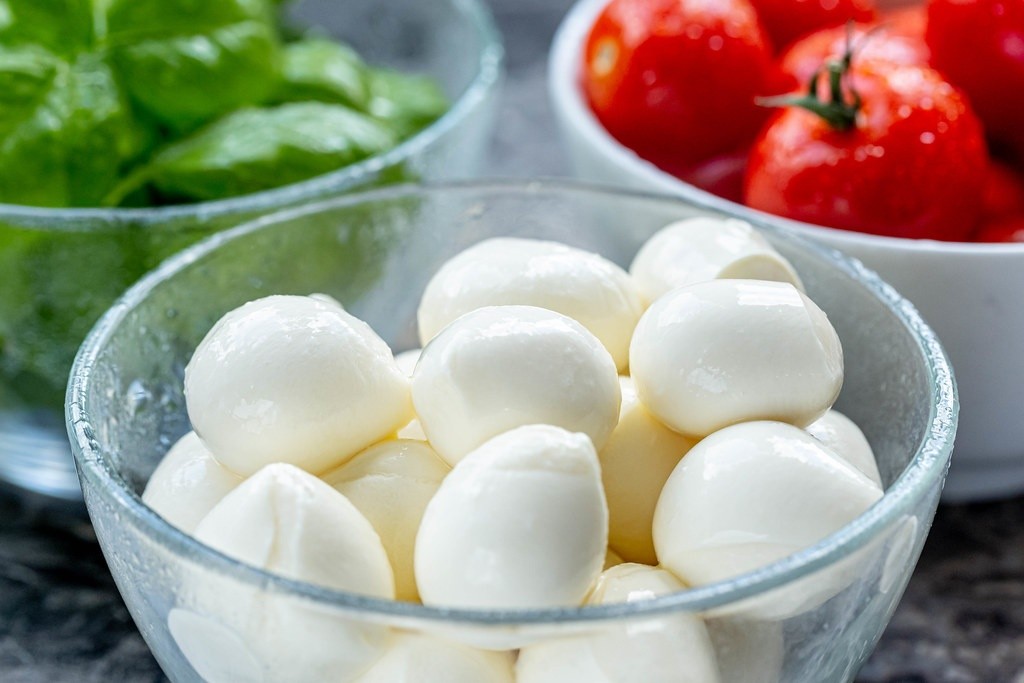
xmin=582 ymin=0 xmax=1024 ymax=245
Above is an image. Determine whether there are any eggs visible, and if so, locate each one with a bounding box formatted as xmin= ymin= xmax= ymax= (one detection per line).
xmin=142 ymin=220 xmax=894 ymax=683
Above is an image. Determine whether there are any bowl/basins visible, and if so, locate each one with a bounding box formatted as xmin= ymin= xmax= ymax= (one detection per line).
xmin=546 ymin=0 xmax=1022 ymax=508
xmin=64 ymin=179 xmax=961 ymax=683
xmin=1 ymin=0 xmax=502 ymax=498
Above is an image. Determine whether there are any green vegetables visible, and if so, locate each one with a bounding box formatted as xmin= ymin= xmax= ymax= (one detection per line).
xmin=1 ymin=0 xmax=456 ymax=429
